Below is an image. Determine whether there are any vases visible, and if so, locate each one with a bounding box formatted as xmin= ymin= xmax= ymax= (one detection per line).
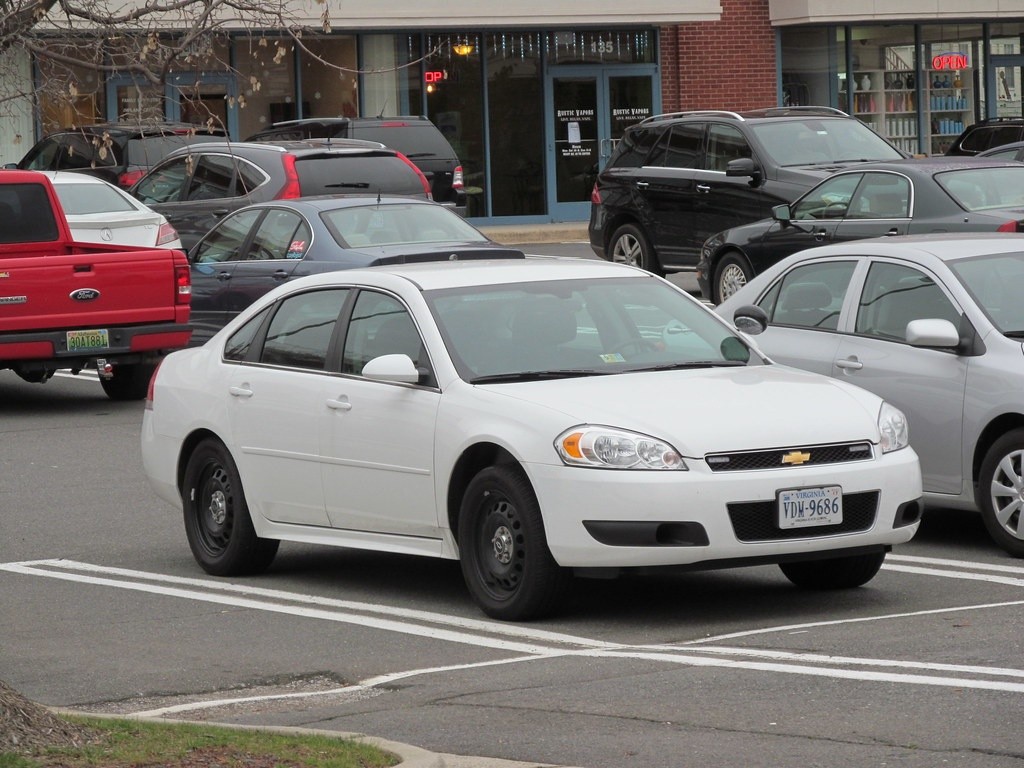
xmin=852 ymin=73 xmax=962 ymax=90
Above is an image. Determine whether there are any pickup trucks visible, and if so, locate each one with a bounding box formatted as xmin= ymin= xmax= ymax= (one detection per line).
xmin=1 ymin=169 xmax=192 ymax=400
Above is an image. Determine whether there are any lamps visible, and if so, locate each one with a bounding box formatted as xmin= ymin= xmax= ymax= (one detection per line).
xmin=453 ymin=38 xmax=474 ymax=56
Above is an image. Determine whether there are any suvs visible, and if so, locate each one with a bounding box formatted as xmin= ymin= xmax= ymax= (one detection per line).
xmin=587 ymin=104 xmax=986 ymax=278
xmin=243 ymin=114 xmax=467 ymax=220
xmin=127 ymin=137 xmax=432 ymax=254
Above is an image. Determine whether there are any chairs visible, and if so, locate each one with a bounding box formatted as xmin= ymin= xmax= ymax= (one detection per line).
xmin=773 ymin=280 xmax=941 ymax=335
xmin=867 ymin=194 xmax=906 ymax=218
xmin=281 ymin=295 xmax=595 ymax=372
xmin=416 ymin=229 xmax=449 ymax=242
xmin=343 ymin=234 xmax=370 ymax=247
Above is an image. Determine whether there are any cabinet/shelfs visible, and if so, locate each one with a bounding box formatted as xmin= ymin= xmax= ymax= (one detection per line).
xmin=853 ymin=67 xmax=975 ymax=158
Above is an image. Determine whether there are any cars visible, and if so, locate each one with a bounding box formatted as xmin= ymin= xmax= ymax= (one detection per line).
xmin=97 ymin=194 xmax=527 ymax=400
xmin=943 ymin=117 xmax=1024 ymax=156
xmin=660 ymin=234 xmax=1024 ymax=560
xmin=140 ymin=262 xmax=924 ymax=621
xmin=696 ymin=155 xmax=1024 ymax=307
xmin=3 ymin=122 xmax=231 ymax=200
xmin=36 ymin=172 xmax=182 ymax=250
